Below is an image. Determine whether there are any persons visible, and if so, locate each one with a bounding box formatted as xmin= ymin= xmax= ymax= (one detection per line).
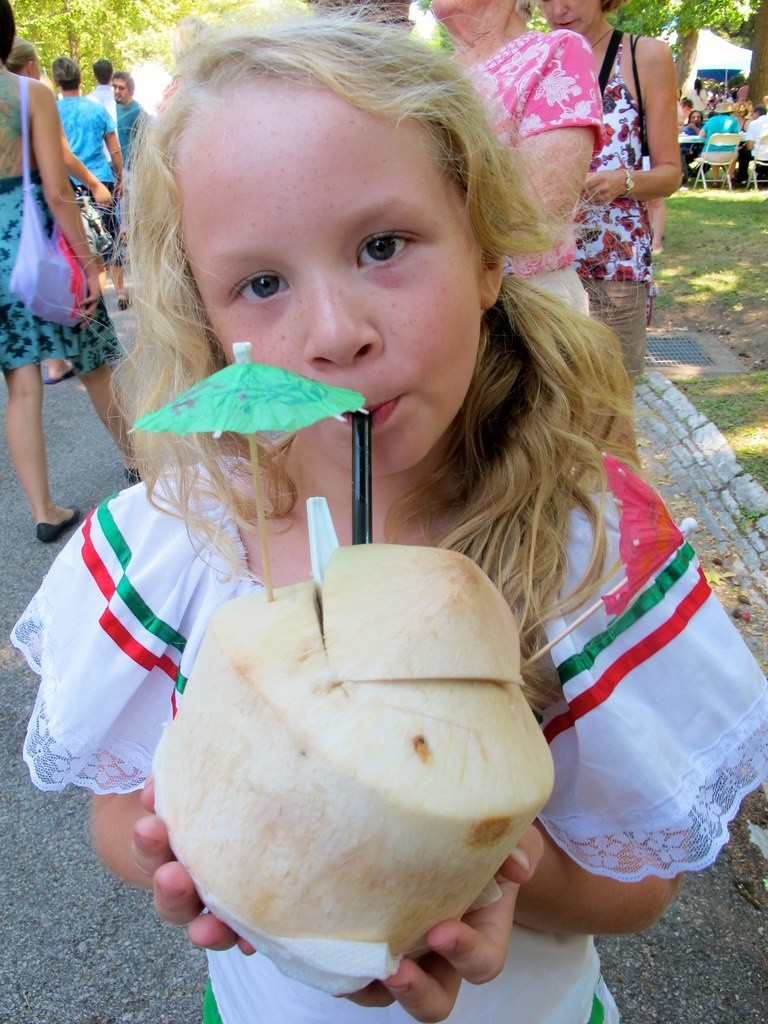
xmin=423 ymin=0 xmax=604 ymax=328
xmin=11 ymin=9 xmax=768 ymax=1024
xmin=542 ymin=0 xmax=685 ymax=460
xmin=53 ymin=56 xmax=133 ymax=311
xmin=107 ymin=72 xmax=147 ymax=249
xmin=677 ymin=77 xmax=768 ymax=189
xmin=83 ymin=60 xmax=122 ymax=165
xmin=4 ymin=35 xmax=113 ymax=385
xmin=1 ymin=0 xmax=147 ymax=544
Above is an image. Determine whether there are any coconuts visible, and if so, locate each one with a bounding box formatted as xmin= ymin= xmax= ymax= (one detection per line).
xmin=154 ymin=542 xmax=555 ymax=989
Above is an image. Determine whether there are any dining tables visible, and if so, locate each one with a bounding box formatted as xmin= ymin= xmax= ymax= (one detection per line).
xmin=678 ymin=133 xmax=752 ymax=184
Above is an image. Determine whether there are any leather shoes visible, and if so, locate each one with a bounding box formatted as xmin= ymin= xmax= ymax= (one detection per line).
xmin=37 ymin=505 xmax=79 ymax=540
xmin=123 ymin=468 xmax=139 ymax=482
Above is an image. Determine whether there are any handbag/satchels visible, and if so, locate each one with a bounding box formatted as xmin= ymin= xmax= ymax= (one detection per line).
xmin=11 ymin=191 xmax=85 ymax=328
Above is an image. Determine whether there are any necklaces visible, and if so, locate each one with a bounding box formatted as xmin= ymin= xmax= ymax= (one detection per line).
xmin=591 ymin=26 xmax=615 ymax=50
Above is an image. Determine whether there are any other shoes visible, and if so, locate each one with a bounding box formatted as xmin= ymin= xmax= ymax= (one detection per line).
xmin=118 ymin=300 xmax=128 ymax=310
xmin=43 ymin=364 xmax=75 ymax=384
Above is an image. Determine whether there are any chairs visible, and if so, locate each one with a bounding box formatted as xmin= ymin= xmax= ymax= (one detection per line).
xmin=746 ymin=134 xmax=768 ymax=191
xmin=691 ymin=133 xmax=741 ymax=192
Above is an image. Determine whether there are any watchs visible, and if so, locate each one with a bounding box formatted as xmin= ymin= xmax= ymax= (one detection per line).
xmin=617 ymin=167 xmax=634 ymax=199
